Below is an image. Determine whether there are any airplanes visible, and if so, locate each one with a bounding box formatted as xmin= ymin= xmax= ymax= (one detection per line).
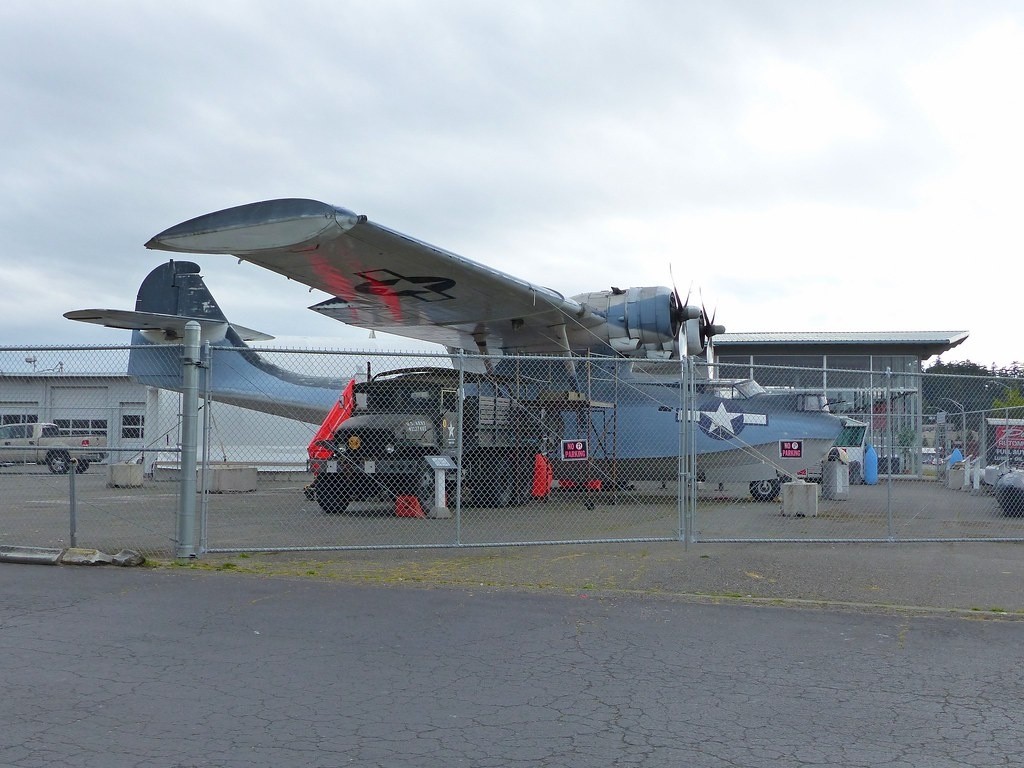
xmin=62 ymin=195 xmax=872 ymax=517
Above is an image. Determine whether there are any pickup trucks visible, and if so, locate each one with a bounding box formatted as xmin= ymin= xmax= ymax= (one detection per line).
xmin=0 ymin=423 xmax=109 ymax=475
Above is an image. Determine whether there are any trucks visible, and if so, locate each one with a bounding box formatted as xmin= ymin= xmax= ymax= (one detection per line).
xmin=302 ymin=366 xmax=554 ymax=510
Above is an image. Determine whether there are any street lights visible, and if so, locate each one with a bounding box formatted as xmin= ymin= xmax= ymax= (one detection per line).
xmin=985 ymin=379 xmax=1012 ymax=462
xmin=939 ymin=396 xmax=967 ymax=460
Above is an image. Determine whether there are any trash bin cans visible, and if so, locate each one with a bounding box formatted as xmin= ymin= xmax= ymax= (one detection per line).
xmin=822 ymin=447 xmax=850 ymax=501
